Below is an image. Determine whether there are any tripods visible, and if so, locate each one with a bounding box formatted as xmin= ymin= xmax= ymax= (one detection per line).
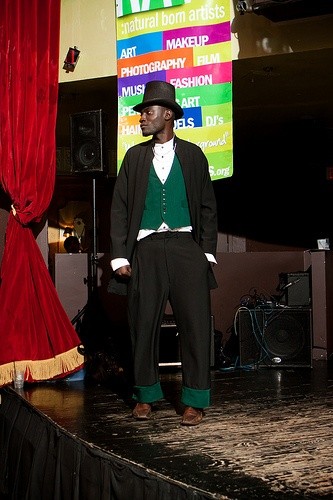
xmin=70 ymin=176 xmax=129 ymax=335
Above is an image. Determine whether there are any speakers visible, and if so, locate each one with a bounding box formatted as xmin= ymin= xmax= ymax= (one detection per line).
xmin=68 ymin=108 xmax=107 ymax=174
xmin=158 ymin=316 xmax=216 ymax=367
xmin=287 ymin=273 xmax=311 ymax=307
xmin=238 ymin=308 xmax=315 ymax=370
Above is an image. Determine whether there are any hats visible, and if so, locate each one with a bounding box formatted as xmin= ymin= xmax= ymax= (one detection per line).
xmin=132 ymin=81 xmax=184 ymax=120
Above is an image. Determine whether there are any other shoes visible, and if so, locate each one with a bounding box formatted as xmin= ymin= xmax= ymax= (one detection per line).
xmin=134 ymin=401 xmax=152 ymax=419
xmin=182 ymin=406 xmax=205 ymax=425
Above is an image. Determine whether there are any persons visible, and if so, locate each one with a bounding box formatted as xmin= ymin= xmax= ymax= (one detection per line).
xmin=64 ymin=236 xmax=80 ymax=253
xmin=109 ymin=80 xmax=218 ymax=425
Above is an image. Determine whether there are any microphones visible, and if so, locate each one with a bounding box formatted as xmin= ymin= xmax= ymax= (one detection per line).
xmin=273 ymin=358 xmax=282 ymax=363
xmin=286 ymin=279 xmax=301 ymax=287
